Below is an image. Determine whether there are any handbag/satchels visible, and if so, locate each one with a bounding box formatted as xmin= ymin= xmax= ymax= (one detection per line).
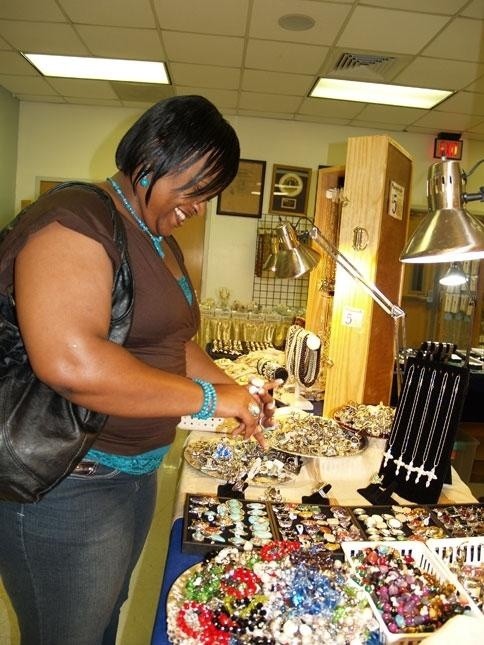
xmin=2 ymin=285 xmax=136 ymax=504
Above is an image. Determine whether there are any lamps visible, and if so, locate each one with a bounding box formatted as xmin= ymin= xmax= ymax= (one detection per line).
xmin=438 ymin=262 xmax=477 ymax=307
xmin=398 ymin=151 xmax=484 ymax=266
xmin=261 ymin=217 xmax=407 ymax=401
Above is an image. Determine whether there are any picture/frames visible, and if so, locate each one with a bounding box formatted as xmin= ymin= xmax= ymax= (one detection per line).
xmin=269 ymin=163 xmax=312 ymax=216
xmin=216 ymin=158 xmax=267 ymax=218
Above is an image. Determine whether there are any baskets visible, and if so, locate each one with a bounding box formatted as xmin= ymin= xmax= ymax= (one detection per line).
xmin=342 ymin=537 xmax=484 ymax=645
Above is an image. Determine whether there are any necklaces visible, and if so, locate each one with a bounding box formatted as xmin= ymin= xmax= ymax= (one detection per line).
xmin=382 ymin=365 xmax=460 ymax=486
xmin=107 ymin=175 xmax=165 ymax=259
xmin=285 ymin=324 xmax=321 ymax=387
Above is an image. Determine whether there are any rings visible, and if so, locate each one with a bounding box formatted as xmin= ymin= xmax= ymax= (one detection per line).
xmin=185 ymin=401 xmax=397 ymax=499
xmin=187 ymin=495 xmax=484 ymax=551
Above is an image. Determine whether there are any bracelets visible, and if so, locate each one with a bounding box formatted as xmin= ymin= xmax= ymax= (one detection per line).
xmin=191 ymin=378 xmax=217 ymax=420
xmin=257 ymin=357 xmax=285 ymax=381
xmin=173 ymin=537 xmax=381 ymax=645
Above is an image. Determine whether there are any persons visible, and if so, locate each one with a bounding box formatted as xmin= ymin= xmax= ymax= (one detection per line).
xmin=0 ymin=95 xmax=265 ymax=645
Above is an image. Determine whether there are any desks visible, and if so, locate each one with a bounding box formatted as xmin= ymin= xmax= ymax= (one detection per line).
xmin=113 ymin=409 xmax=484 ymax=645
